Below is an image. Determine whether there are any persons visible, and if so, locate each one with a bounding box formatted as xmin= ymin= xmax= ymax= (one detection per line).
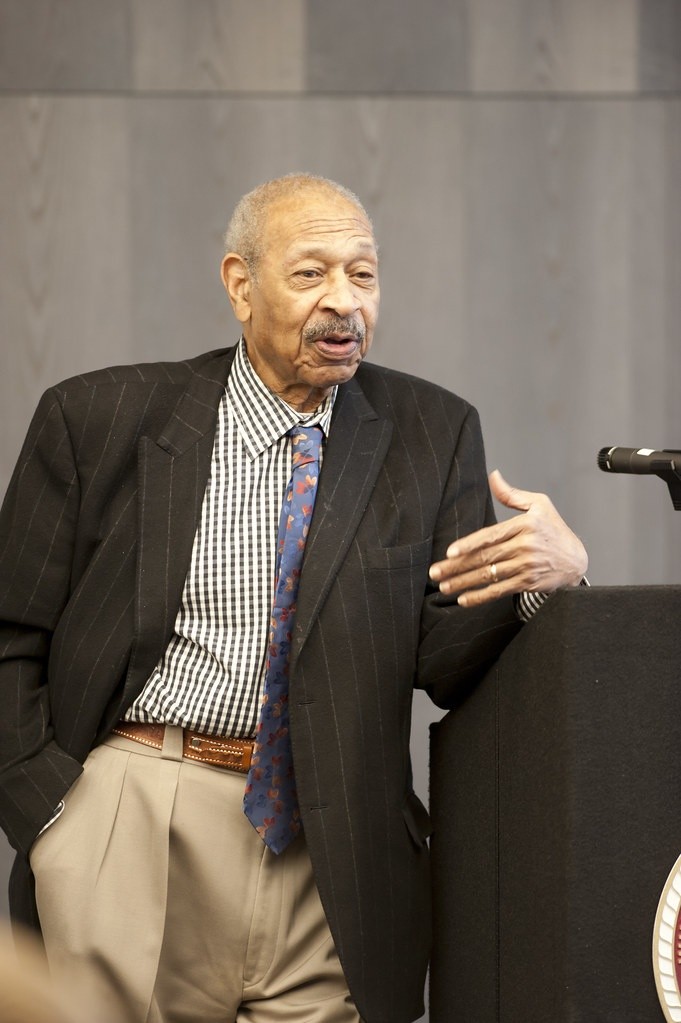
xmin=1 ymin=173 xmax=595 ymax=1023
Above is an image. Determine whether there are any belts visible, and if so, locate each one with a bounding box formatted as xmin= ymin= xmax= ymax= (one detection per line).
xmin=112 ymin=715 xmax=255 ymax=772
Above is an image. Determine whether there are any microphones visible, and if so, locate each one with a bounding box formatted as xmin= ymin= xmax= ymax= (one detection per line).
xmin=596 ymin=446 xmax=681 ymax=476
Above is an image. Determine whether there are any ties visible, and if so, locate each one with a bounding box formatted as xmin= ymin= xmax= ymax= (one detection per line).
xmin=242 ymin=423 xmax=327 ymax=852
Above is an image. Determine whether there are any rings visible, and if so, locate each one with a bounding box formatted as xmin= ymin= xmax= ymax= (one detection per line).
xmin=491 ymin=563 xmax=498 ymax=582
